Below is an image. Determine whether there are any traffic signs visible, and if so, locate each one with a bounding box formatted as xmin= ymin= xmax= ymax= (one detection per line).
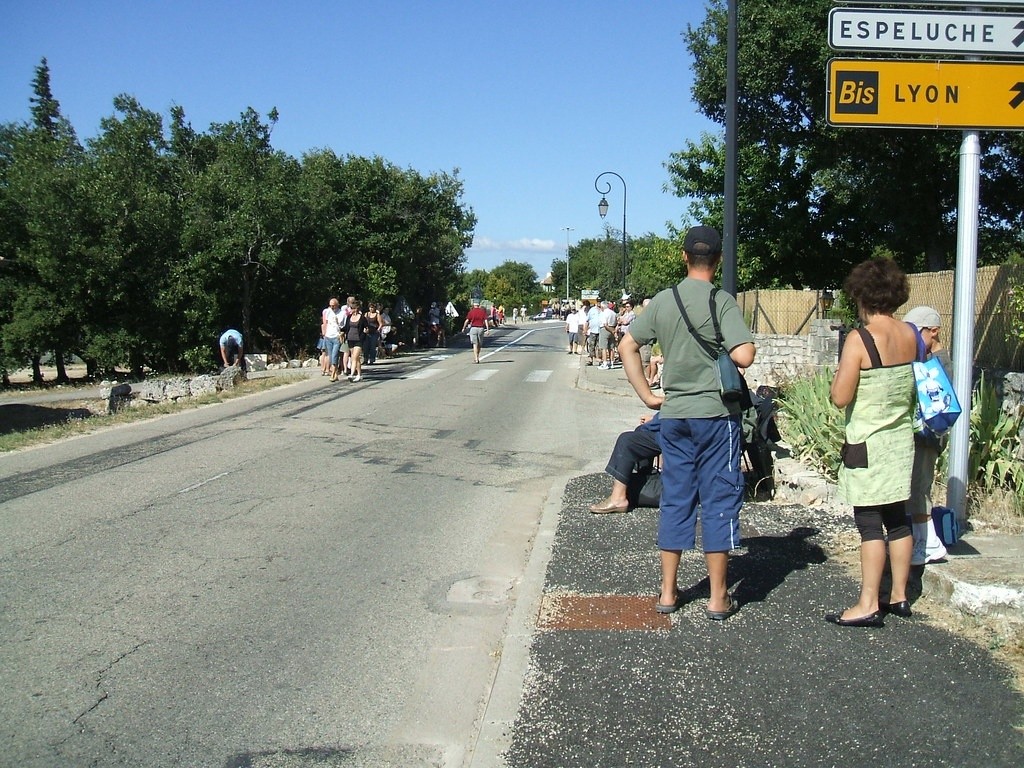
xmin=827 ymin=6 xmax=1024 ymax=59
xmin=826 ymin=55 xmax=1024 ymax=133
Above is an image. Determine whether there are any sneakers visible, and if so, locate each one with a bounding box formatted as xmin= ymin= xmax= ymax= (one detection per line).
xmin=906 ymin=539 xmax=948 ymax=566
xmin=596 ymin=364 xmax=609 ymax=370
xmin=607 ymin=363 xmax=614 ymax=369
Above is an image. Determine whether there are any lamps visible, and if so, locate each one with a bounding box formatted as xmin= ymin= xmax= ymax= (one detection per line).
xmin=818 ymin=292 xmax=836 ymax=318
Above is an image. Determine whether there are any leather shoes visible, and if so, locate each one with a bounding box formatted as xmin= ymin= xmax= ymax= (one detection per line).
xmin=825 ymin=609 xmax=885 ymax=628
xmin=877 ymin=600 xmax=913 ymax=617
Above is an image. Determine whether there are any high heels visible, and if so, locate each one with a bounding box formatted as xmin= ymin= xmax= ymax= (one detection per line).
xmin=589 ymin=496 xmax=629 ymax=514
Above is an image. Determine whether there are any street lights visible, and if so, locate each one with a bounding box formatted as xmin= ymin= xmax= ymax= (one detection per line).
xmin=561 ymin=227 xmax=575 ymax=304
xmin=594 ymin=172 xmax=627 ymax=289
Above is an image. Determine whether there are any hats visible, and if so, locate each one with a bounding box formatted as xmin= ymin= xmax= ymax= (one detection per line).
xmin=608 ymin=303 xmax=615 ymax=309
xmin=596 ymin=297 xmax=602 ymax=302
xmin=684 ymin=226 xmax=722 ymax=255
xmin=430 ymin=302 xmax=437 ymax=308
xmin=473 ymin=302 xmax=480 ymax=306
xmin=900 ymin=306 xmax=941 ymax=332
xmin=571 ymin=305 xmax=576 ymax=310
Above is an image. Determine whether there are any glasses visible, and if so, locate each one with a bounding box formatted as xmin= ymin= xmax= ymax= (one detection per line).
xmin=625 ymin=306 xmax=631 ymax=308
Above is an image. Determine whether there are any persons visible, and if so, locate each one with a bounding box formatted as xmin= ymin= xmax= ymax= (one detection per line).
xmin=219 ymin=329 xmax=246 ymax=372
xmin=462 ymin=299 xmax=489 ymax=363
xmin=566 ymin=296 xmax=664 ymax=387
xmin=520 ymin=305 xmax=527 ymax=322
xmin=317 ymin=296 xmax=402 ymax=382
xmin=589 ymin=412 xmax=659 ymax=515
xmin=491 ymin=304 xmax=506 ymax=327
xmin=428 ymin=302 xmax=446 ymax=347
xmin=886 ymin=306 xmax=953 ymax=569
xmin=617 ymin=226 xmax=755 ymax=621
xmin=513 ymin=308 xmax=518 ymax=324
xmin=825 ymin=255 xmax=927 ymax=628
xmin=553 ymin=301 xmax=570 ymax=321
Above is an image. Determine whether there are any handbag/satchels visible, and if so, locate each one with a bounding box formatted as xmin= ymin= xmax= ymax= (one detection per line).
xmin=904 ymin=322 xmax=962 ymax=439
xmin=626 ymin=455 xmax=665 ymax=508
xmin=339 ymin=338 xmax=350 ymax=353
xmin=316 ymin=335 xmax=326 ymax=350
xmin=708 ymin=288 xmax=753 ymax=410
xmin=903 ymin=505 xmax=961 ymax=547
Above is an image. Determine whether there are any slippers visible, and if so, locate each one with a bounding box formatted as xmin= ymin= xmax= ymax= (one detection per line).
xmin=705 ymin=594 xmax=740 ymax=620
xmin=656 ymin=588 xmax=686 ymax=613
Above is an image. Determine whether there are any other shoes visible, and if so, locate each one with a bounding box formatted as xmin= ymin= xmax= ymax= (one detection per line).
xmin=600 ymin=361 xmax=603 ymax=366
xmin=585 ymin=360 xmax=592 ymax=366
